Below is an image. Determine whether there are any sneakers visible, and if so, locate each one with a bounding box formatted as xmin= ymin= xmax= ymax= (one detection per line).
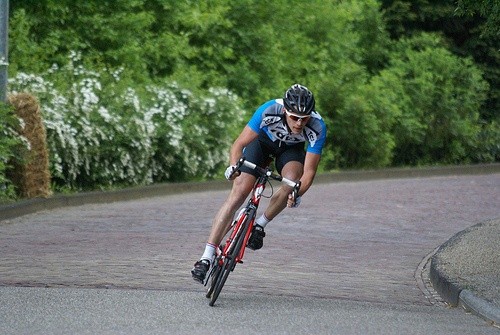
xmin=249 ymin=224 xmax=265 ymax=249
xmin=190 ymin=259 xmax=210 ymax=279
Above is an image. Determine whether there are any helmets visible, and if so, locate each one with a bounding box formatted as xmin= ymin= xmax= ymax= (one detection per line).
xmin=284 ymin=84 xmax=315 ymax=115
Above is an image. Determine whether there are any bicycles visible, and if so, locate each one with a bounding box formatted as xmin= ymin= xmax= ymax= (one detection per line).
xmin=194 ymin=156 xmax=302 ymax=307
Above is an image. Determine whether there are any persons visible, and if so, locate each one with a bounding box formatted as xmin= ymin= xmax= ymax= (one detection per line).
xmin=191 ymin=84 xmax=326 ymax=280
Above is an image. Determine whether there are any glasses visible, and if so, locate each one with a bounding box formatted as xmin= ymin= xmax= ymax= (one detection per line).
xmin=285 ymin=109 xmax=312 ymax=121
